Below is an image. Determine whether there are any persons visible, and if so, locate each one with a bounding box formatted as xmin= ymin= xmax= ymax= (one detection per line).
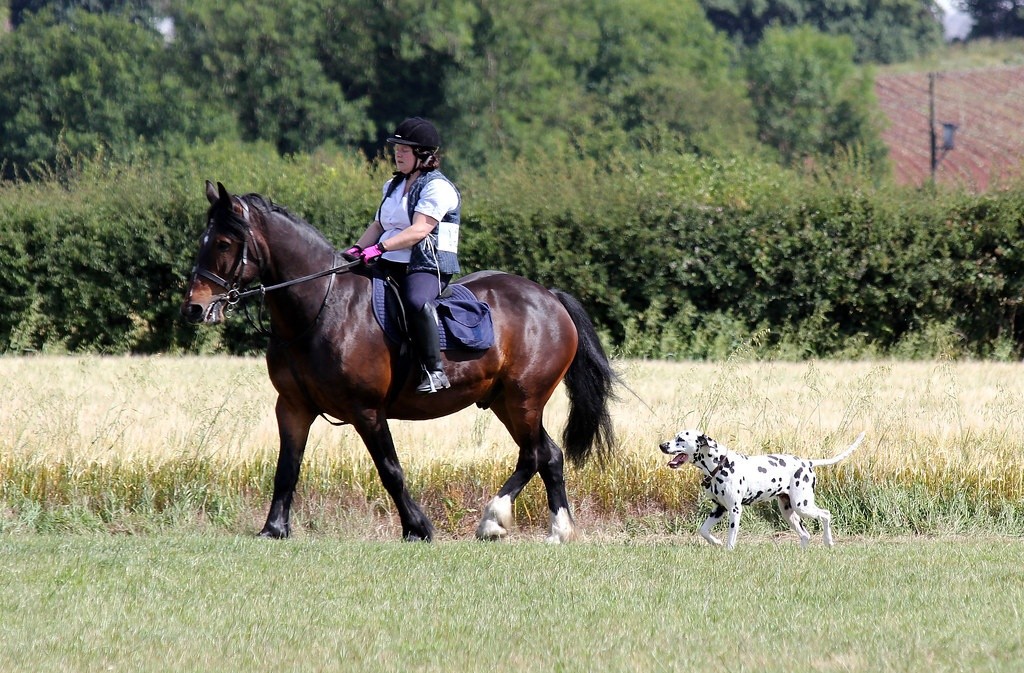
xmin=343 ymin=116 xmax=463 ymax=393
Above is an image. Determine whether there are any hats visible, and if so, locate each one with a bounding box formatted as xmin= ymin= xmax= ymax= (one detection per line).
xmin=386 ymin=116 xmax=439 ymax=149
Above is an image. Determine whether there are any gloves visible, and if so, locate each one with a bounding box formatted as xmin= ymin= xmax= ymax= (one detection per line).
xmin=339 ymin=244 xmax=362 ymax=262
xmin=359 ymin=242 xmax=387 ymax=268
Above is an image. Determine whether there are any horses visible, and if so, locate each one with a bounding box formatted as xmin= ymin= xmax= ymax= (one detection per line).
xmin=178 ymin=180 xmax=657 ymax=541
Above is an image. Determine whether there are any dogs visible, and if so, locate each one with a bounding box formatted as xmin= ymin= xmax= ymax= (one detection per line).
xmin=659 ymin=430 xmax=868 ymax=551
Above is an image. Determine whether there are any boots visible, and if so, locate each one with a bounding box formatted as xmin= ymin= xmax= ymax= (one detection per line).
xmin=415 ymin=301 xmax=451 ymax=395
